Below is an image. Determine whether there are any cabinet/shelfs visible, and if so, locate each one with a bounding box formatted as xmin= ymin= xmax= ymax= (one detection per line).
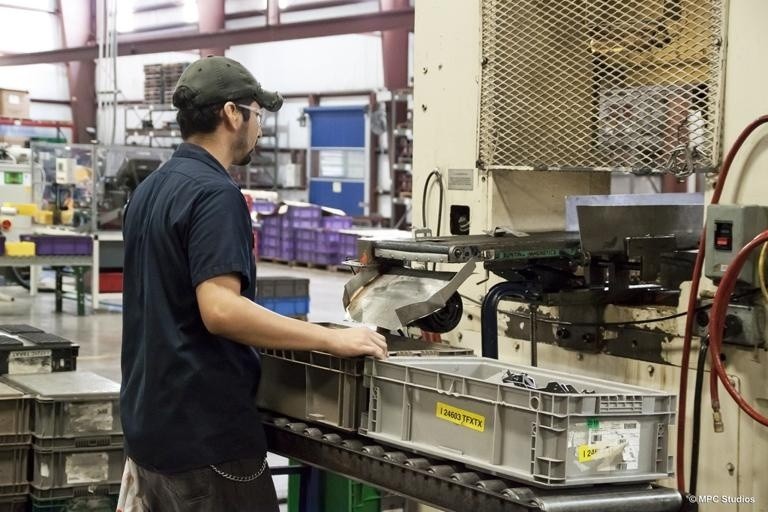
xmin=389 ymin=88 xmax=412 ymax=231
xmin=124 ymin=102 xmax=278 ymax=191
xmin=252 ymin=197 xmax=375 ymax=322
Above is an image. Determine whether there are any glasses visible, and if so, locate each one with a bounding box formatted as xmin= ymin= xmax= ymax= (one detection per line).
xmin=236 ymin=99 xmax=269 ymax=123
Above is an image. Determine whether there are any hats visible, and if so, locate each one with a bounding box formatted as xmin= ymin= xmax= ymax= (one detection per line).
xmin=173 ymin=54 xmax=284 ymax=113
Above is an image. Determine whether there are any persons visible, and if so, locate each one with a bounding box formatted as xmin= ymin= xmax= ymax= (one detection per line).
xmin=113 ymin=54 xmax=388 ymax=512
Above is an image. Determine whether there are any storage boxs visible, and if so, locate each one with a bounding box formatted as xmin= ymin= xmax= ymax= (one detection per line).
xmin=83 ymin=272 xmax=123 ymax=293
xmin=20 ymin=234 xmax=93 ymax=256
xmin=0 ymin=87 xmax=30 ymax=120
xmin=358 ymin=355 xmax=677 ymax=488
xmin=256 ymin=322 xmax=474 ymax=433
xmin=144 ymin=62 xmax=189 ymax=104
xmin=0 ymin=324 xmax=127 ymax=512
